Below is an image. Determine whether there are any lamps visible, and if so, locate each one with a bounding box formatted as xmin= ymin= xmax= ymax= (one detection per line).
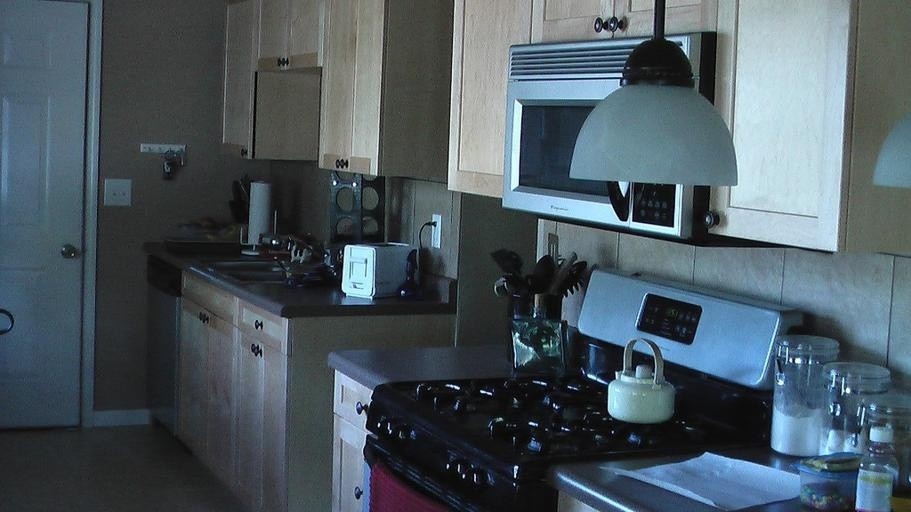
xmin=558 ymin=4 xmax=741 ymax=193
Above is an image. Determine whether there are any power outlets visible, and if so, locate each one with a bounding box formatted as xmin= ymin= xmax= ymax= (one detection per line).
xmin=430 ymin=212 xmax=442 ymax=250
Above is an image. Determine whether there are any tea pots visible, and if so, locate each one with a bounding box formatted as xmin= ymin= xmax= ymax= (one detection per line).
xmin=605 ymin=336 xmax=678 ymax=425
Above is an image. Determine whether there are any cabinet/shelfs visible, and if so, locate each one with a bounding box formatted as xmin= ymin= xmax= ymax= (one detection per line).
xmin=173 ymin=263 xmax=237 ymax=503
xmin=220 ymin=0 xmax=322 ymax=166
xmin=704 ymin=0 xmax=910 ymax=260
xmin=328 ymin=364 xmax=379 ymax=511
xmin=528 ymin=2 xmax=720 ymax=48
xmin=442 ymin=0 xmax=536 ymax=201
xmin=555 ymin=489 xmax=599 ymax=511
xmin=255 ymin=0 xmax=326 ymax=78
xmin=230 ymin=292 xmax=456 ymax=512
xmin=316 ymin=0 xmax=455 ymax=186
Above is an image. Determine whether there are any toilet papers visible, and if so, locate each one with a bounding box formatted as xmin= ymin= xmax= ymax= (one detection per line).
xmin=247 ymin=181 xmax=274 ymax=244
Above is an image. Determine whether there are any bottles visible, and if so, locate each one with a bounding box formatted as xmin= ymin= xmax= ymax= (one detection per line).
xmin=771 ymin=334 xmax=909 ymax=511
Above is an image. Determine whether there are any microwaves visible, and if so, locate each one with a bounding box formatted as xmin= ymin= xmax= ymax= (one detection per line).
xmin=499 ymin=32 xmax=769 ymax=249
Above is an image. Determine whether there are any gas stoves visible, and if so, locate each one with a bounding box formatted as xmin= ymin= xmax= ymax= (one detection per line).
xmin=364 ymin=379 xmax=739 ymax=510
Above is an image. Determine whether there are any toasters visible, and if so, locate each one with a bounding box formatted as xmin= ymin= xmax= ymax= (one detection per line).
xmin=340 ymin=240 xmax=413 ymax=302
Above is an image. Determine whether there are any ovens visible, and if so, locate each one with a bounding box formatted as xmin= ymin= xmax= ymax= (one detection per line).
xmin=364 ymin=435 xmax=536 ymax=512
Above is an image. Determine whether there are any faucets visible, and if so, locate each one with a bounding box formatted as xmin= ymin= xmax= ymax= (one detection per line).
xmin=274 ymin=256 xmax=288 ymax=272
xmin=287 ymin=232 xmax=313 ymax=254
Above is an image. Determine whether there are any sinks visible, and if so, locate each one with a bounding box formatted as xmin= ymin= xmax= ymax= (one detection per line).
xmin=235 ymin=272 xmax=287 ymax=282
xmin=234 ymin=260 xmax=282 ymax=272
